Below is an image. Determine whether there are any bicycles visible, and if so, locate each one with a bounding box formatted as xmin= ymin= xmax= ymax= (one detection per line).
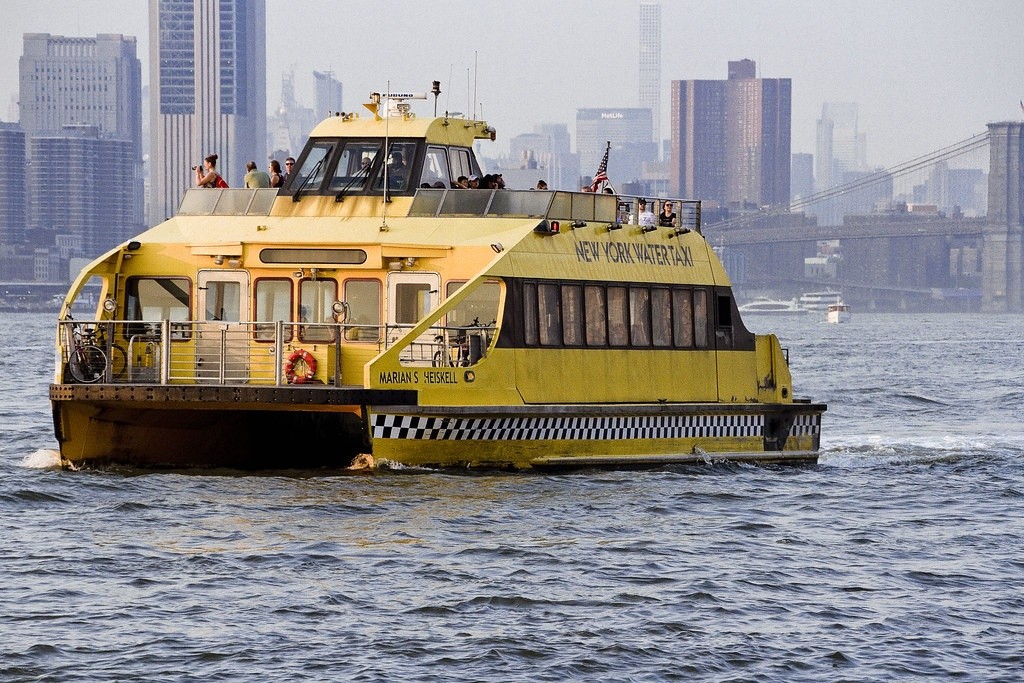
xmin=62 ymin=311 xmax=128 ymax=384
xmin=430 ymin=316 xmax=497 ymax=368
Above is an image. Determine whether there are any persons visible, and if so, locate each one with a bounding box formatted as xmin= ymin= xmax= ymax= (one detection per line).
xmin=244 ymin=162 xmax=272 ymax=188
xmin=451 ymin=173 xmax=613 ymax=194
xmin=616 ymin=197 xmax=677 ymax=227
xmin=196 ymin=154 xmax=218 ymax=188
xmin=387 ymin=152 xmax=406 ymax=177
xmin=268 ymin=160 xmax=284 ymax=188
xmin=282 ymin=157 xmax=301 ymax=188
xmin=351 ymin=157 xmax=372 ymax=178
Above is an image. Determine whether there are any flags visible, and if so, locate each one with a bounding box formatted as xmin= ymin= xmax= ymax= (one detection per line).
xmin=589 ymin=148 xmax=609 ymax=193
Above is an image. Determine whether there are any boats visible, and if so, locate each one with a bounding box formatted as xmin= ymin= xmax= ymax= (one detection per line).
xmin=795 ymin=285 xmax=843 ymax=312
xmin=736 ymin=294 xmax=810 ymax=316
xmin=46 ymin=50 xmax=830 ymax=475
xmin=825 ymin=296 xmax=852 ymax=324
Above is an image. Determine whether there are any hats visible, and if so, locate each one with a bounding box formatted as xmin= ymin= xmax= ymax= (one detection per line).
xmin=467 ymin=174 xmax=478 ymax=182
xmin=431 ymin=181 xmax=446 ymax=187
xmin=639 ymin=197 xmax=645 ymax=201
xmin=493 ymin=174 xmax=502 ymax=180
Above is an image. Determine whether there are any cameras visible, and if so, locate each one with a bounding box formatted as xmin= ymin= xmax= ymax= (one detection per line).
xmin=192 ymin=166 xmax=203 ymax=171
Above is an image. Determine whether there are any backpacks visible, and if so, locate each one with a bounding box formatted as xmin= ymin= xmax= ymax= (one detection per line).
xmin=212 ymin=172 xmax=229 ymax=188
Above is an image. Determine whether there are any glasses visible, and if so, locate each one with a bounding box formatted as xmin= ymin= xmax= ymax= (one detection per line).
xmin=286 ymin=162 xmax=294 ymax=166
xmin=640 ymin=202 xmax=646 ymax=205
xmin=665 ymin=203 xmax=673 ymax=206
xmin=540 ymin=188 xmax=548 ymax=190
xmin=362 ymin=162 xmax=369 ymax=165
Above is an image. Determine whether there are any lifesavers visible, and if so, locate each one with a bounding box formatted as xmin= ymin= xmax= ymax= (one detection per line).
xmin=285 ymin=350 xmax=318 ymax=384
